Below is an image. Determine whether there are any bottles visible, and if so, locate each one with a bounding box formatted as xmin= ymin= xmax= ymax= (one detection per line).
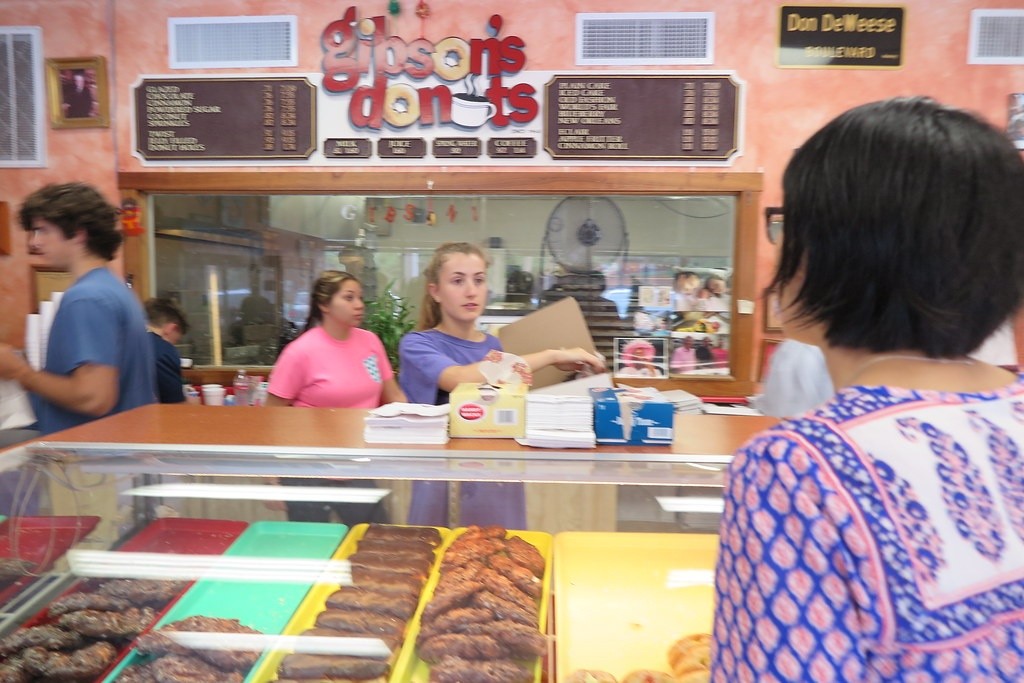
xmin=233 ymin=368 xmax=250 ymax=407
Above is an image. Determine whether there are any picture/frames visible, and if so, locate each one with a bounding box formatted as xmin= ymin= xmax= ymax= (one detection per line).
xmin=611 ymin=335 xmax=669 ymax=382
xmin=47 ymin=54 xmax=110 ymax=130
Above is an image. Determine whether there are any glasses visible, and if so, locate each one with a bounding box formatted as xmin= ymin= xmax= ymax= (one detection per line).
xmin=765 ymin=204 xmax=788 ymax=247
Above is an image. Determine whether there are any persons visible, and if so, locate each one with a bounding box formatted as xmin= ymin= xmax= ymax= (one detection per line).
xmin=669 ymin=331 xmax=698 ymax=373
xmin=702 ymin=274 xmax=732 ymax=312
xmin=61 ymin=68 xmax=93 ymax=119
xmin=261 ymin=267 xmax=409 ymax=525
xmin=701 ymin=92 xmax=1024 ymax=683
xmin=397 ymin=239 xmax=607 ymax=529
xmin=141 ymin=294 xmax=191 ymax=404
xmin=694 ymin=337 xmax=714 ymax=369
xmin=709 ymin=335 xmax=731 ymax=369
xmin=618 ymin=346 xmax=663 ymax=377
xmin=0 ymin=179 xmax=161 ymax=435
xmin=688 ymin=287 xmax=714 ymax=311
xmin=644 ymin=268 xmax=701 ymax=310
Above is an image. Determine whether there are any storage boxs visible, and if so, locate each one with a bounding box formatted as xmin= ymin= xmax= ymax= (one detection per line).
xmin=389 ymin=528 xmax=552 ymax=682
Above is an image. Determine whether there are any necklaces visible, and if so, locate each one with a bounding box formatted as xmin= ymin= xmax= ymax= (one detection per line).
xmin=837 ymin=345 xmax=981 ymax=379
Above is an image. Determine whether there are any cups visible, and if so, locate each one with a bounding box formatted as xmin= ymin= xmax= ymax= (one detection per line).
xmin=180 ymin=383 xmax=226 ymax=406
xmin=23 ymin=288 xmax=65 ymax=376
